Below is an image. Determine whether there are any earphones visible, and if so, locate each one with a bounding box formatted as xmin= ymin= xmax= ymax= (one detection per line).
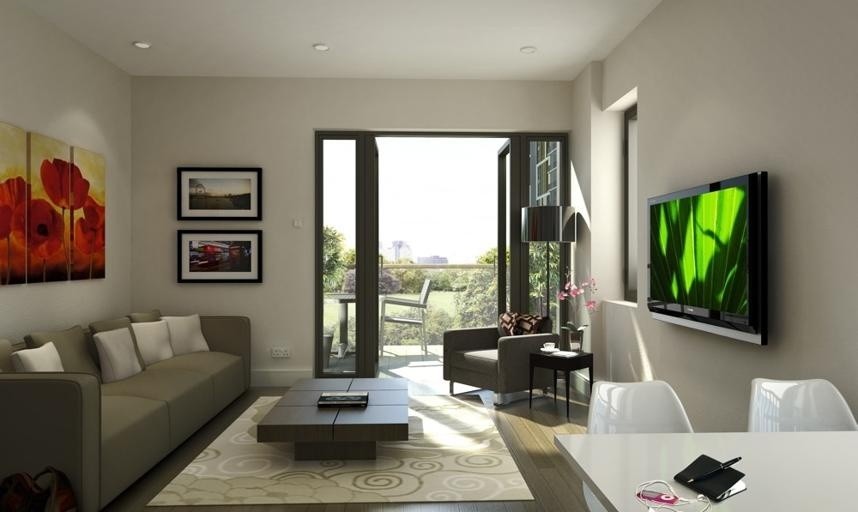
xmin=694 ymin=493 xmax=707 ymax=502
xmin=648 ymin=506 xmax=654 ymax=512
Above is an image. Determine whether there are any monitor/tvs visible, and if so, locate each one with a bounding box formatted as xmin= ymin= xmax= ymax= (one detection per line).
xmin=647 ymin=170 xmax=769 ymax=345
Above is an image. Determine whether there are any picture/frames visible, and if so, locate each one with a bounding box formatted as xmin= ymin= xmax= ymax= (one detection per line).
xmin=176 ymin=230 xmax=262 ymax=283
xmin=177 ymin=167 xmax=262 ymax=220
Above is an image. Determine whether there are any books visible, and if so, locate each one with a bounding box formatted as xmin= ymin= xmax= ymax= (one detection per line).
xmin=673 ymin=454 xmax=747 ymax=502
xmin=317 ymin=391 xmax=369 ymax=409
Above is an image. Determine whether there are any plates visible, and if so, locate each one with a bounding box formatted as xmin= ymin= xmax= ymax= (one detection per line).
xmin=539 ymin=348 xmax=559 ymax=354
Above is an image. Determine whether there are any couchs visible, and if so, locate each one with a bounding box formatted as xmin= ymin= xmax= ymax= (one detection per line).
xmin=0 ymin=316 xmax=250 ymax=512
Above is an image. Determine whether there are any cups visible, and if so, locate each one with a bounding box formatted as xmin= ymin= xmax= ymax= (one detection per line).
xmin=542 ymin=342 xmax=555 ymax=349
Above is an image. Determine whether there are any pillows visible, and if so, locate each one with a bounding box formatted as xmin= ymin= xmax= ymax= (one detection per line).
xmin=128 ymin=320 xmax=173 ymax=366
xmin=24 ymin=325 xmax=103 ymax=384
xmin=0 ymin=338 xmax=15 ymax=370
xmin=89 ymin=317 xmax=145 ymax=372
xmin=10 ymin=342 xmax=64 ymax=373
xmin=160 ymin=313 xmax=209 ymax=355
xmin=126 ymin=309 xmax=160 ymax=323
xmin=93 ymin=327 xmax=141 ymax=383
xmin=499 ymin=312 xmax=540 ymax=335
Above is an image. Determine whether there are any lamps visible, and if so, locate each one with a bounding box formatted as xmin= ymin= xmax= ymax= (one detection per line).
xmin=521 ymin=206 xmax=575 ymax=317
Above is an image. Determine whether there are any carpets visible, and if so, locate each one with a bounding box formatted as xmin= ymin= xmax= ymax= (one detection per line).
xmin=146 ymin=394 xmax=534 ymax=507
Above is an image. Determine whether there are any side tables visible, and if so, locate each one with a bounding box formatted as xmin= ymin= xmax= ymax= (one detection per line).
xmin=529 ymin=350 xmax=593 ymax=418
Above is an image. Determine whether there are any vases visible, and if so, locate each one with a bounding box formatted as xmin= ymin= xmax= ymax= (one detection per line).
xmin=569 ymin=325 xmax=582 ymax=351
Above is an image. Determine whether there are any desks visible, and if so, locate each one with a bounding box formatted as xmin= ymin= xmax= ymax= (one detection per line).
xmin=553 ymin=431 xmax=858 ymax=512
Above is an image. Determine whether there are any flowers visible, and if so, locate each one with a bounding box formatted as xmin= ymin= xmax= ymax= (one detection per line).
xmin=557 ymin=277 xmax=598 ymax=324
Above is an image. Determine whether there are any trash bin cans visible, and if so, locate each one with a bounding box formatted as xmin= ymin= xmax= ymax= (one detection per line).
xmin=323 ymin=335 xmax=333 ymax=368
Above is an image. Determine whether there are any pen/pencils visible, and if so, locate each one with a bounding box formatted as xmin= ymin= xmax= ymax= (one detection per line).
xmin=687 ymin=457 xmax=742 ymax=483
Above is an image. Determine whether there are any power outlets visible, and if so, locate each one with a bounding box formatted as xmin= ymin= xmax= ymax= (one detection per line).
xmin=271 ymin=346 xmax=289 ymax=358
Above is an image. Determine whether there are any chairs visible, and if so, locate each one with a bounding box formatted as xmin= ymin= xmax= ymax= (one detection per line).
xmin=444 ymin=317 xmax=554 ymax=406
xmin=582 ymin=380 xmax=694 ymax=512
xmin=748 ymin=378 xmax=858 ymax=432
xmin=377 ymin=279 xmax=431 ymax=357
